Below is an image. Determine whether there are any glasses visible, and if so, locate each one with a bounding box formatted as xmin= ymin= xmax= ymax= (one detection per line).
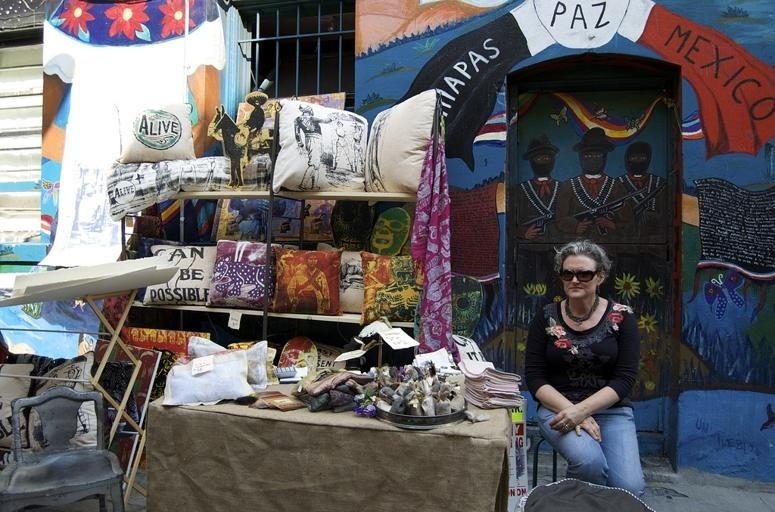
xmin=558 ymin=269 xmax=602 ymax=283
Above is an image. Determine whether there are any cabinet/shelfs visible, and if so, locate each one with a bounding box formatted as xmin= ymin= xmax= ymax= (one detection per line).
xmin=110 ymin=90 xmax=450 ymax=463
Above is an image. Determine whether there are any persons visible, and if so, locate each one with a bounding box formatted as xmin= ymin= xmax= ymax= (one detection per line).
xmin=526 ymin=241 xmax=646 ymax=501
xmin=558 ymin=128 xmax=632 ymax=302
xmin=333 ymin=120 xmax=354 ymax=171
xmin=371 ymin=208 xmax=410 ymax=255
xmin=287 ymin=251 xmax=330 ymax=315
xmin=331 ymin=198 xmax=372 ymax=254
xmin=512 ymin=135 xmax=564 ymax=325
xmin=351 ymin=122 xmax=366 ymax=174
xmin=293 ymin=105 xmax=339 ymax=192
xmin=241 ymin=91 xmax=268 ymax=162
xmin=42 ymin=0 xmax=226 ymax=268
xmin=620 ymin=141 xmax=673 ymax=323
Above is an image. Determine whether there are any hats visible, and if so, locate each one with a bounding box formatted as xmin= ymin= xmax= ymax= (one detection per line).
xmin=302 ymin=251 xmax=325 ymax=257
xmin=521 ymin=134 xmax=561 ymax=161
xmin=244 ymin=91 xmax=268 ymax=104
xmin=574 ymin=125 xmax=613 ymax=153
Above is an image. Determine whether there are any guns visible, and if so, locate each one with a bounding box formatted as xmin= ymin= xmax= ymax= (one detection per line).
xmin=632 ymin=180 xmax=669 ymax=218
xmin=569 ymin=187 xmax=648 ymax=237
xmin=520 ymin=213 xmax=554 ymax=237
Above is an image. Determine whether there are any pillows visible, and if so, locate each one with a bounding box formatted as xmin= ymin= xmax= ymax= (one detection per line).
xmin=215 ymin=238 xmax=269 ymax=268
xmin=365 ymin=89 xmax=436 ymax=191
xmin=273 ymin=97 xmax=368 ymax=194
xmin=144 ymin=241 xmax=215 ymax=306
xmin=271 ymin=244 xmax=342 ymax=313
xmin=359 ymin=250 xmax=421 ymax=325
xmin=112 ymin=96 xmax=194 ymax=163
xmin=164 ymin=351 xmax=255 ymax=407
xmin=105 ymin=157 xmax=234 ymax=221
xmin=317 ymin=241 xmax=365 ymax=314
xmin=186 ymin=336 xmax=267 ymax=390
xmin=207 ymin=258 xmax=274 ymax=312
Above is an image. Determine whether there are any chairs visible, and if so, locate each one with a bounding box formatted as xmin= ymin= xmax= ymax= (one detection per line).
xmin=0 ymin=385 xmax=125 ymax=511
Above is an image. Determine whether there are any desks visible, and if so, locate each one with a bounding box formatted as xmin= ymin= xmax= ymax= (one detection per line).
xmin=145 ymin=376 xmax=509 ymax=512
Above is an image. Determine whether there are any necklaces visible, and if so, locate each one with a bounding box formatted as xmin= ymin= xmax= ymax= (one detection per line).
xmin=564 ymin=293 xmax=599 ymax=326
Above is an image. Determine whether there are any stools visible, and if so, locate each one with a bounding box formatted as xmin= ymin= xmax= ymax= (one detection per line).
xmin=530 ymin=430 xmax=558 ymax=488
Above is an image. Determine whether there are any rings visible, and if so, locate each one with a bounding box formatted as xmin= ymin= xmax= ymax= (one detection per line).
xmin=562 ymin=425 xmax=568 ymax=433
